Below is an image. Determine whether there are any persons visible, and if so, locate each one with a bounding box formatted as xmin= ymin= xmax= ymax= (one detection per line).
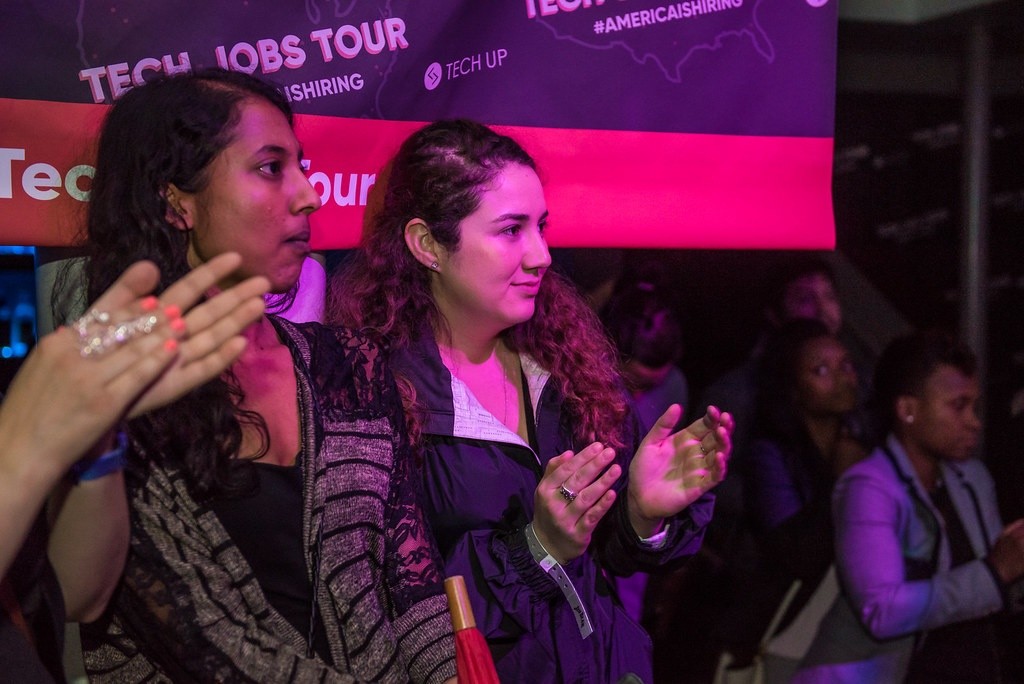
xmin=358 ymin=121 xmax=738 ymax=684
xmin=0 ymin=243 xmax=279 ymax=683
xmin=570 ymin=236 xmax=1024 ymax=684
xmin=77 ymin=70 xmax=456 ymax=683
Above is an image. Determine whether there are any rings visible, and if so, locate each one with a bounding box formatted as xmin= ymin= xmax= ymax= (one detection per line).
xmin=558 ymin=482 xmax=577 ymax=501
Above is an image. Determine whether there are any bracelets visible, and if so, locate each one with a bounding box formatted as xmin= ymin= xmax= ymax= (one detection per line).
xmin=70 ymin=429 xmax=130 ymax=481
xmin=523 ymin=519 xmax=593 ymax=640
